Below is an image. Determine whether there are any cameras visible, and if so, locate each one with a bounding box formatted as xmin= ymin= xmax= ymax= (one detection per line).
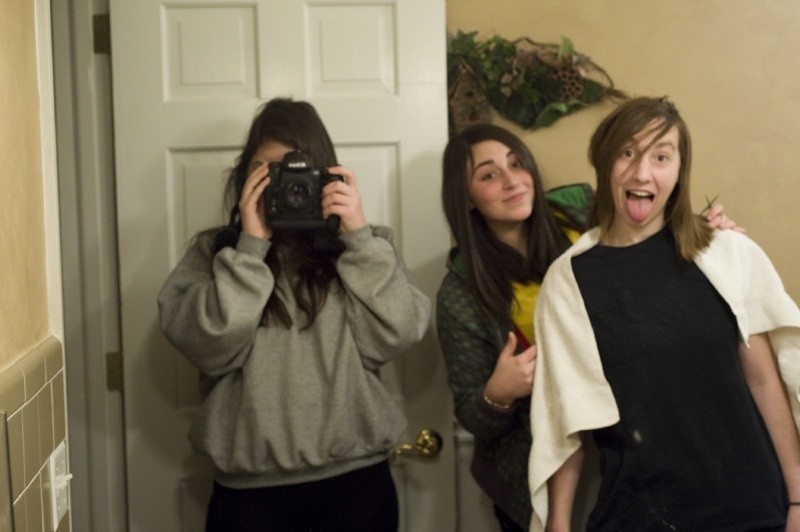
xmin=261 ymin=151 xmax=342 ymax=229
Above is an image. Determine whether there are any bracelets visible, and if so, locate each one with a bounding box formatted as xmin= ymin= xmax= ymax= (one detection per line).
xmin=788 ymin=502 xmax=800 ymax=505
xmin=484 ymin=397 xmax=509 ymax=411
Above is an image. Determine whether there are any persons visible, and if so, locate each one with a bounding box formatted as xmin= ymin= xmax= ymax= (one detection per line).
xmin=529 ymin=95 xmax=800 ymax=532
xmin=433 ymin=122 xmax=747 ymax=532
xmin=156 ymin=98 xmax=430 ymax=532
